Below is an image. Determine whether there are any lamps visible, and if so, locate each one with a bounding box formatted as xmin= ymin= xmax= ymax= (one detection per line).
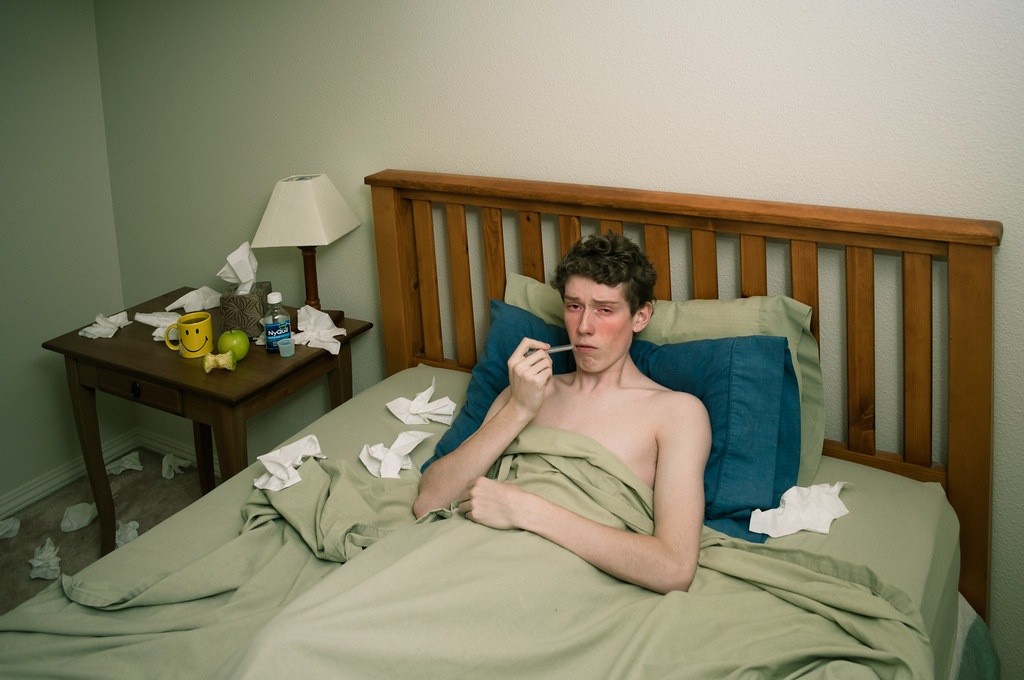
xmin=250 ymin=173 xmax=362 ymax=334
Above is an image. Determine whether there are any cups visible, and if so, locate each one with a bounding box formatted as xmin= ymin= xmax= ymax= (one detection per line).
xmin=164 ymin=312 xmax=214 ymax=359
xmin=277 ymin=338 xmax=294 ymax=356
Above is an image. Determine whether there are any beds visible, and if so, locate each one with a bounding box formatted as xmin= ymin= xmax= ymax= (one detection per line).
xmin=0 ymin=168 xmax=1005 ymax=680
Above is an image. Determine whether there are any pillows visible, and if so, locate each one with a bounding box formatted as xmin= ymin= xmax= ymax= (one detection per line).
xmin=504 ymin=272 xmax=826 ymax=488
xmin=419 ymin=298 xmax=801 ymax=543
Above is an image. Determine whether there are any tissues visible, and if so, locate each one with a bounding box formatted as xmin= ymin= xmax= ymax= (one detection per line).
xmin=214 ymin=242 xmax=273 ymax=342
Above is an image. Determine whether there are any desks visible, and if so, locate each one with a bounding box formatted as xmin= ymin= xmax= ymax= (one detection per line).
xmin=42 ymin=287 xmax=375 ymax=559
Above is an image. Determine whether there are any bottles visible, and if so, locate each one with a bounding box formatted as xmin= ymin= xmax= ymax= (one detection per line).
xmin=264 ymin=292 xmax=291 ymax=353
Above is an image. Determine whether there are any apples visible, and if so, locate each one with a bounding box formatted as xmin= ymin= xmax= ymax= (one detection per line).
xmin=217 ymin=326 xmax=250 ymax=362
xmin=203 ymin=350 xmax=236 ymax=374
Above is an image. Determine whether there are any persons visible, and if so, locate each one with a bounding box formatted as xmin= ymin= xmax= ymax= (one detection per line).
xmin=231 ymin=227 xmax=712 ymax=680
